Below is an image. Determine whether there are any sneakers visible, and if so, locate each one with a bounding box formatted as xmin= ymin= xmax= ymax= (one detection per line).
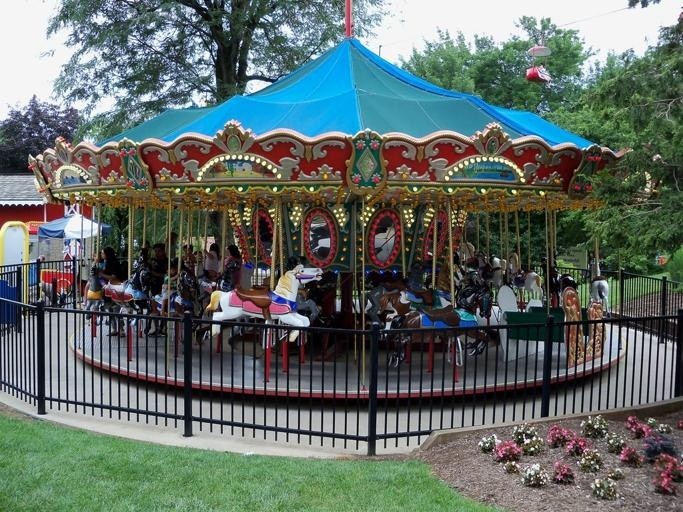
xmin=148 ymin=328 xmax=166 ymax=337
xmin=106 ymin=332 xmax=125 ymax=337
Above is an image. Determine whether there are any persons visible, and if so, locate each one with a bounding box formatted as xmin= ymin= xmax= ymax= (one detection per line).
xmin=81 ymin=232 xmax=243 ymax=339
xmin=27 ymin=254 xmax=45 ymax=270
